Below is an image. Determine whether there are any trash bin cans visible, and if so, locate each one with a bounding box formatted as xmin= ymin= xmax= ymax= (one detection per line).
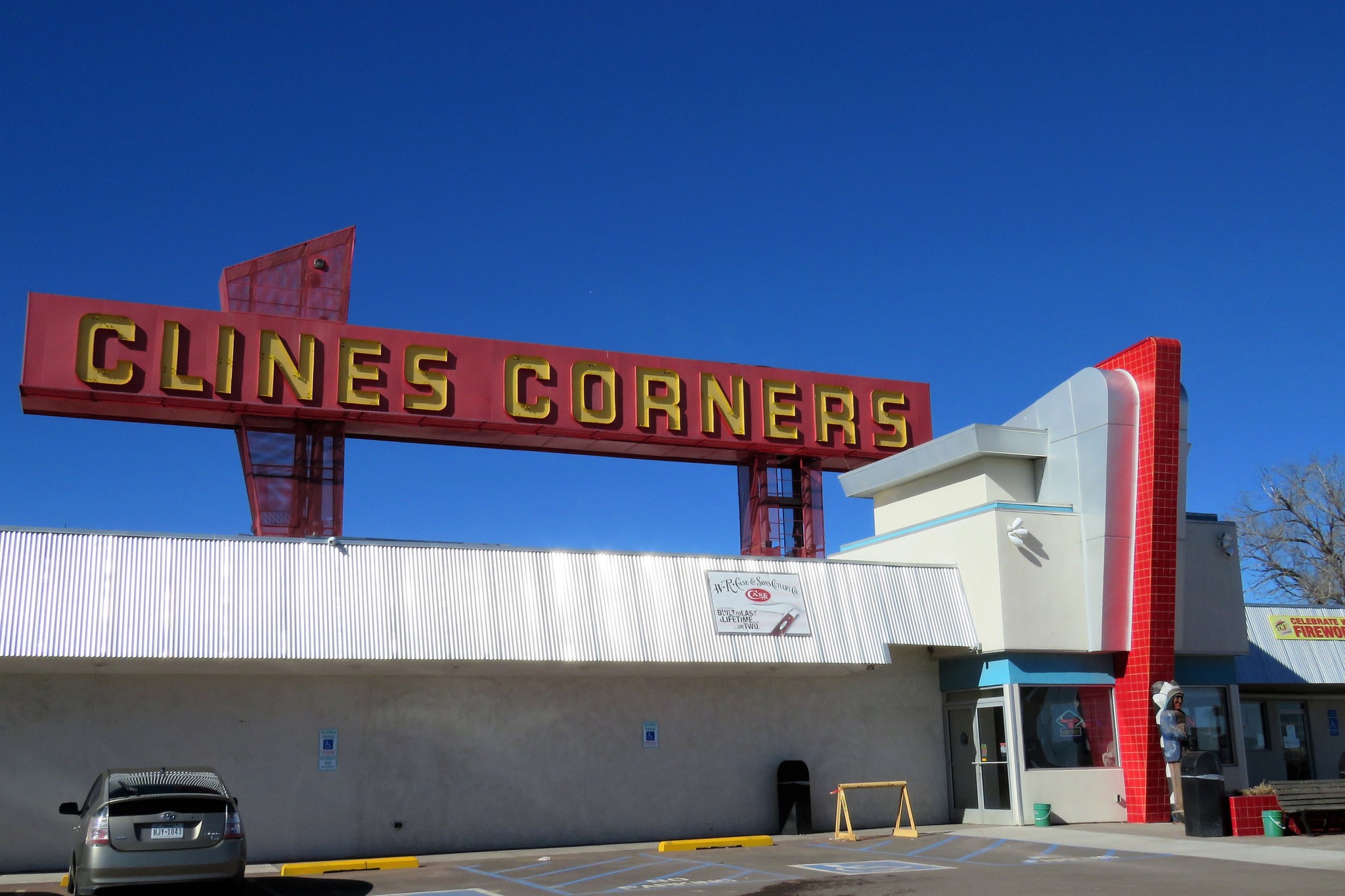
xmin=1181 ymin=750 xmax=1228 ymax=838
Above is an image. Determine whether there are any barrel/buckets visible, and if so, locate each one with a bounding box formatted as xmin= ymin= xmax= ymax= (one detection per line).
xmin=1033 ymin=803 xmax=1051 ymax=826
xmin=1261 ymin=810 xmax=1285 ymax=837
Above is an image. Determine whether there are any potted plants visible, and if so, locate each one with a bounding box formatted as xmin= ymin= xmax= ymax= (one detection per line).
xmin=1228 ymin=778 xmax=1301 ymax=838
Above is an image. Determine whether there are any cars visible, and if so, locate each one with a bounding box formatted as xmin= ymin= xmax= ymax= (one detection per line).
xmin=58 ymin=766 xmax=248 ymax=896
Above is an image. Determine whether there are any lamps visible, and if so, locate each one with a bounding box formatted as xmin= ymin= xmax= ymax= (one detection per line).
xmin=1007 ymin=517 xmax=1028 ymax=547
xmin=1219 ymin=532 xmax=1233 ymax=557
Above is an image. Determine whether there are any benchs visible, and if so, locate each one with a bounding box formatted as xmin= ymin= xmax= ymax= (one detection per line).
xmin=1268 ymin=779 xmax=1345 ymax=837
